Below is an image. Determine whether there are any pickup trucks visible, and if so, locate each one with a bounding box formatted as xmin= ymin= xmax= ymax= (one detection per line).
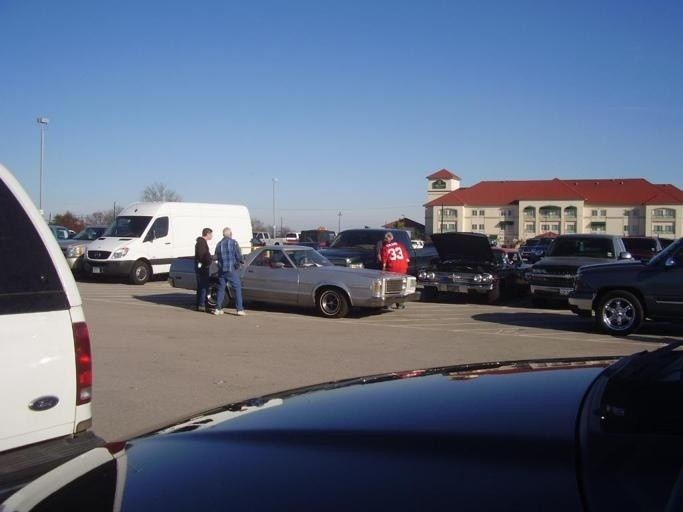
xmin=316 ymin=229 xmax=439 ymax=275
xmin=166 ymin=244 xmax=418 ymax=319
xmin=252 ymin=229 xmax=335 ymax=249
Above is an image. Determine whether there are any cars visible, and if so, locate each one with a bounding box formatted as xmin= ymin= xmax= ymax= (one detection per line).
xmin=416 ymin=231 xmax=534 ymax=302
xmin=409 ymin=240 xmax=423 ymax=249
xmin=526 ymin=233 xmax=632 ymax=303
xmin=0 ymin=339 xmax=682 ymax=512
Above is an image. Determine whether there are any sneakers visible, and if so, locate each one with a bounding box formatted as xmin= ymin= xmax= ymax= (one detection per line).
xmin=396 ymin=305 xmax=406 ymax=309
xmin=214 ymin=309 xmax=224 ymax=315
xmin=238 ymin=311 xmax=248 ymax=315
xmin=199 ymin=307 xmax=212 ymax=312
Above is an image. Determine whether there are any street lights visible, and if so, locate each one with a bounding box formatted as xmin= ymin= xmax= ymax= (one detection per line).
xmin=271 ymin=177 xmax=278 ymax=238
xmin=36 ymin=118 xmax=48 ymax=216
xmin=337 ymin=211 xmax=342 ymax=234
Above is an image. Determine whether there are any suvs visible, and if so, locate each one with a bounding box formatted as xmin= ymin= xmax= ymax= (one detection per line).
xmin=519 ymin=237 xmax=554 ymax=260
xmin=567 ymin=236 xmax=682 ymax=337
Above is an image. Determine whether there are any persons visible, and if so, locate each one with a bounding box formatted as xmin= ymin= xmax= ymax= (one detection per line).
xmin=270 ymin=250 xmax=286 ymax=268
xmin=300 ymin=251 xmax=316 ymax=265
xmin=380 ymin=232 xmax=410 ymax=274
xmin=194 ymin=228 xmax=213 ymax=312
xmin=211 ymin=227 xmax=246 ymax=316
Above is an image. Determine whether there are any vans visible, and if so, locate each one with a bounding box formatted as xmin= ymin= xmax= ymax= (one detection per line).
xmin=0 ymin=164 xmax=105 ymax=503
xmin=47 ymin=224 xmax=107 ymax=278
xmin=0 ymin=440 xmax=132 ymax=511
xmin=619 ymin=236 xmax=675 ymax=266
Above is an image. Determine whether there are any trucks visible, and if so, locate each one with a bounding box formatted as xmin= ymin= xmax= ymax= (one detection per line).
xmin=84 ymin=200 xmax=253 ymax=286
xmin=152 ymin=396 xmax=284 ymax=435
